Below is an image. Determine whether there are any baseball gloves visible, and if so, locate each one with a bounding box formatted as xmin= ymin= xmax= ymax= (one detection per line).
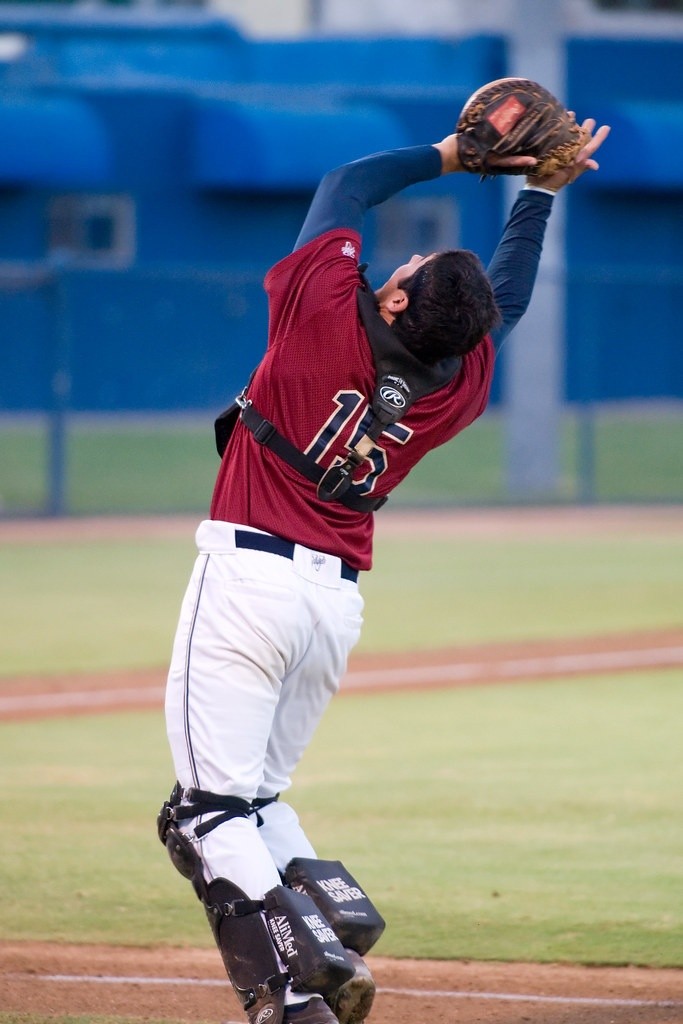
xmin=454 ymin=77 xmax=593 ymax=180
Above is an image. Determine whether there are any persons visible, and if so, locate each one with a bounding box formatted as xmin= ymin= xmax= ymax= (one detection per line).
xmin=156 ymin=89 xmax=613 ymax=1024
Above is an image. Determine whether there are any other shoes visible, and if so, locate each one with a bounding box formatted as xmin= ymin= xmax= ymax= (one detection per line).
xmin=282 ymin=997 xmax=339 ymax=1024
xmin=324 ymin=949 xmax=375 ymax=1024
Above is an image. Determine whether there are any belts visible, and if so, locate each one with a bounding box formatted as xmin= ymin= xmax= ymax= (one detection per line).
xmin=235 ymin=530 xmax=359 ymax=583
